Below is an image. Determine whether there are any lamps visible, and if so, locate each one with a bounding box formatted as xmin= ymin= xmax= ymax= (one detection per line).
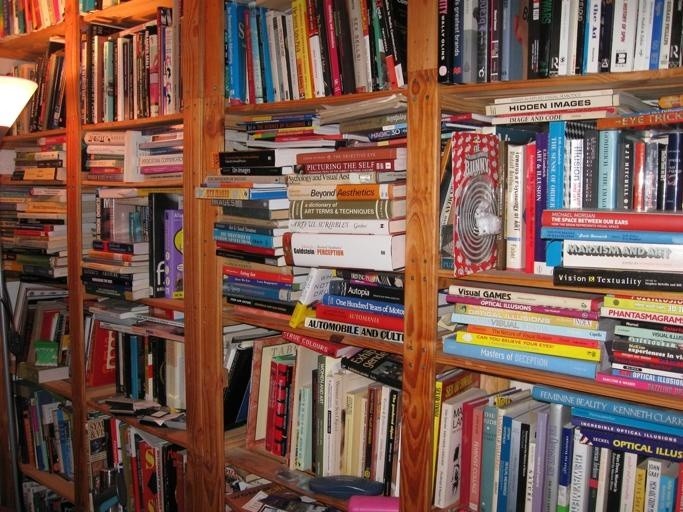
xmin=0 ymin=76 xmax=38 ymax=138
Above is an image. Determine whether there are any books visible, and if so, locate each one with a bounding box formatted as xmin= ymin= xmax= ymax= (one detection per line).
xmin=79 ymin=121 xmax=186 ymax=186
xmin=85 ymin=407 xmax=187 ymax=511
xmin=225 ymin=467 xmax=335 ymax=511
xmin=80 ymin=184 xmax=186 ymax=295
xmin=0 ymin=40 xmax=71 ymax=138
xmin=224 ymin=1 xmax=406 ymax=90
xmin=222 ymin=321 xmax=403 ymax=495
xmin=437 ymin=1 xmax=680 ymax=83
xmin=433 ymin=91 xmax=682 ymax=273
xmin=1 ymin=2 xmax=69 ymax=36
xmin=4 ymin=286 xmax=72 ymax=385
xmin=82 ymin=296 xmax=186 ymax=409
xmin=433 ymin=274 xmax=682 ymax=363
xmin=0 ymin=140 xmax=70 ymax=285
xmin=9 ymin=387 xmax=76 ymax=485
xmin=188 ymin=93 xmax=411 ymax=322
xmin=15 ymin=475 xmax=74 ymax=511
xmin=75 ymin=0 xmax=183 ymax=120
xmin=430 ymin=363 xmax=681 ymax=509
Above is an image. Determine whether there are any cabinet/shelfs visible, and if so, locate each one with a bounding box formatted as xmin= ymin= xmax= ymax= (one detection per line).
xmin=200 ymin=0 xmax=416 ymax=512
xmin=0 ymin=0 xmax=72 ymax=512
xmin=425 ymin=0 xmax=683 ymax=512
xmin=71 ymin=0 xmax=200 ymax=512
